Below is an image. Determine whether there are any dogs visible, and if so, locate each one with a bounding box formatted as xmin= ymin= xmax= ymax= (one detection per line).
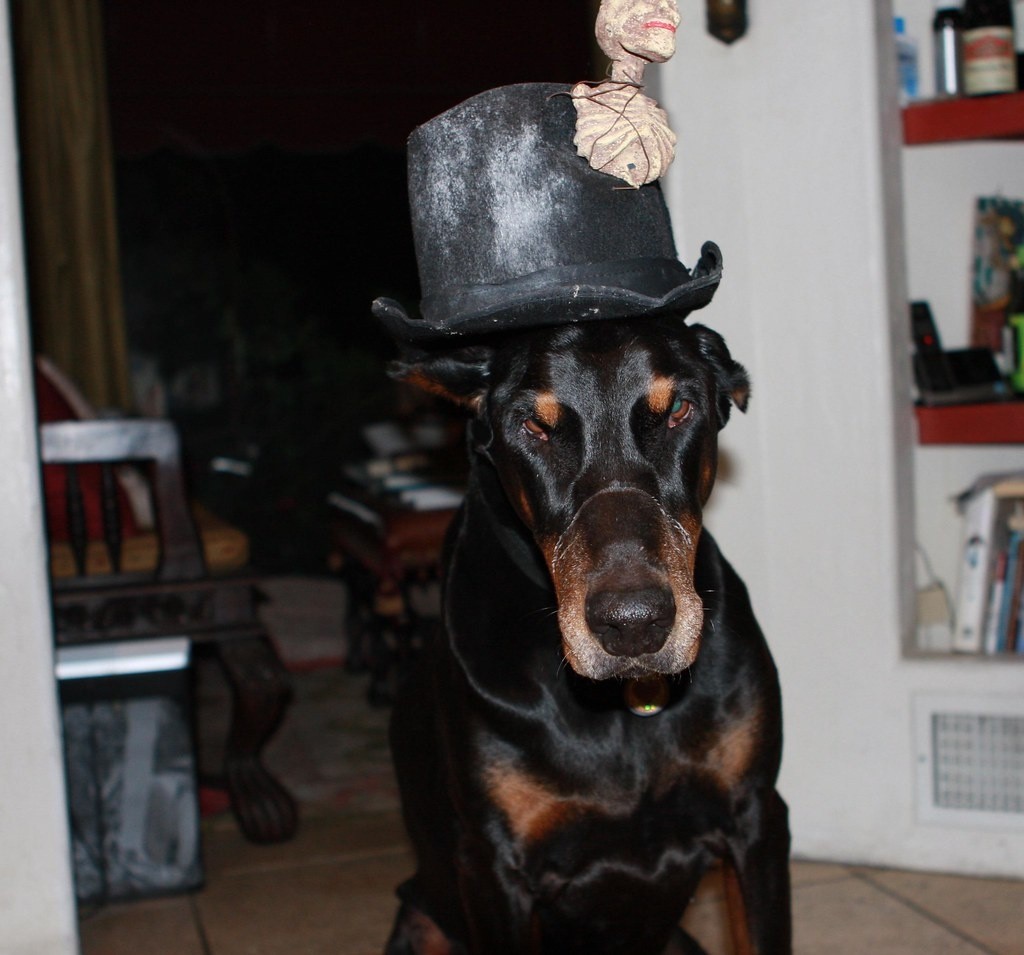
xmin=381 ymin=314 xmax=796 ymax=955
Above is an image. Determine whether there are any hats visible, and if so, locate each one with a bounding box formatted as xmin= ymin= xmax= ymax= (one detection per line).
xmin=371 ymin=82 xmax=723 ymax=334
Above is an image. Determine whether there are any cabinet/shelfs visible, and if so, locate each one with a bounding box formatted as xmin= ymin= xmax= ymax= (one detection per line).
xmin=870 ymin=0 xmax=1023 ymax=666
